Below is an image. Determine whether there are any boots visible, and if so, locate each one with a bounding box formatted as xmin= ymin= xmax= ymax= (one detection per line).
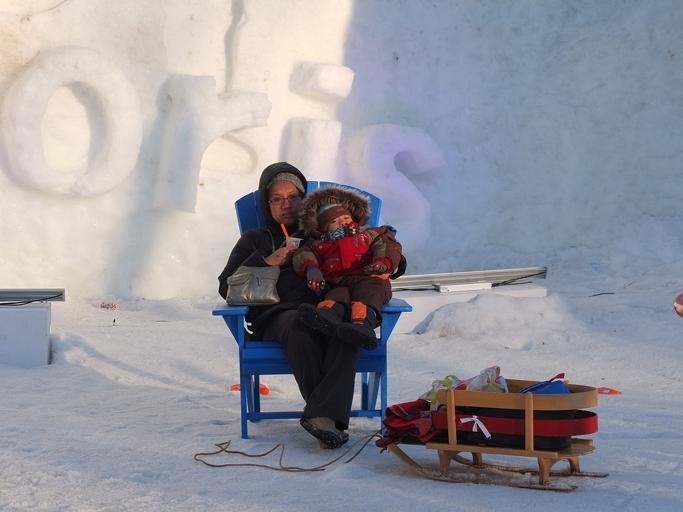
xmin=297 ymin=299 xmax=377 ymax=351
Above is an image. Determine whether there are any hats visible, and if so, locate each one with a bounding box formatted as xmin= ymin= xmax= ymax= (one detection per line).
xmin=267 ymin=173 xmax=305 ymax=193
xmin=316 ymin=203 xmax=351 ymax=233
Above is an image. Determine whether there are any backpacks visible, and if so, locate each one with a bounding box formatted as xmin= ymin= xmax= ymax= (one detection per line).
xmin=519 ymin=373 xmax=574 ymax=394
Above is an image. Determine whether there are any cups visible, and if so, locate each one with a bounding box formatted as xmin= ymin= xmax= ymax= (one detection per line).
xmin=286 ymin=237 xmax=304 ymax=248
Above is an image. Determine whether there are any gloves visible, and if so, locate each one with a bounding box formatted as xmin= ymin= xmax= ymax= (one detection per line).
xmin=306 ymin=265 xmax=325 ymax=292
xmin=334 ymin=269 xmax=365 ymax=288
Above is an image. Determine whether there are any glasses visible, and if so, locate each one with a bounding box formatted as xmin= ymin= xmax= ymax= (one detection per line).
xmin=267 ymin=194 xmax=301 ymax=205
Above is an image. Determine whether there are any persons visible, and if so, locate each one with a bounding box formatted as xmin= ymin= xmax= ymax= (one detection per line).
xmin=288 ymin=185 xmax=403 ymax=324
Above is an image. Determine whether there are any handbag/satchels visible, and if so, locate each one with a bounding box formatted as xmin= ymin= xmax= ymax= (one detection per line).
xmin=226 ymin=228 xmax=280 ymax=306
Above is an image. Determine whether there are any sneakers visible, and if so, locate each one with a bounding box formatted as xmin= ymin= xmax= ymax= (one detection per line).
xmin=299 ymin=416 xmax=349 ymax=450
